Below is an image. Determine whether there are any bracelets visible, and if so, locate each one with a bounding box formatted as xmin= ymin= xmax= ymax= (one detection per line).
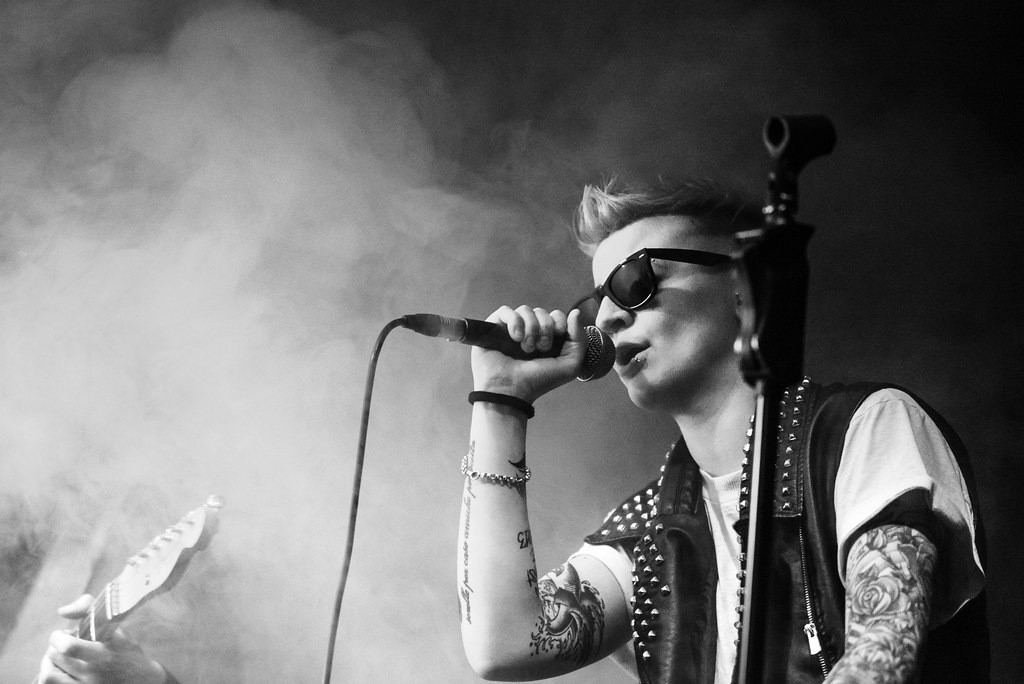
xmin=461 ymin=455 xmax=532 ymax=487
xmin=468 ymin=391 xmax=535 ymax=419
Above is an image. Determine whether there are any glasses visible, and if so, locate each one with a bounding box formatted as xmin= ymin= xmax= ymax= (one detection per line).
xmin=567 ymin=245 xmax=735 ymax=327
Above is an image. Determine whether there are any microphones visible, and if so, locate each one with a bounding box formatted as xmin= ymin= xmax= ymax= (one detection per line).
xmin=400 ymin=314 xmax=617 ymax=383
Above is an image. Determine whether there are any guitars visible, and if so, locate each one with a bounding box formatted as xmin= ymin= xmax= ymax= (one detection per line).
xmin=30 ymin=492 xmax=226 ymax=684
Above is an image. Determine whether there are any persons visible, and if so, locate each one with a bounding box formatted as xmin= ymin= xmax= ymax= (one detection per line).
xmin=0 ymin=492 xmax=179 ymax=684
xmin=457 ymin=173 xmax=985 ymax=684
